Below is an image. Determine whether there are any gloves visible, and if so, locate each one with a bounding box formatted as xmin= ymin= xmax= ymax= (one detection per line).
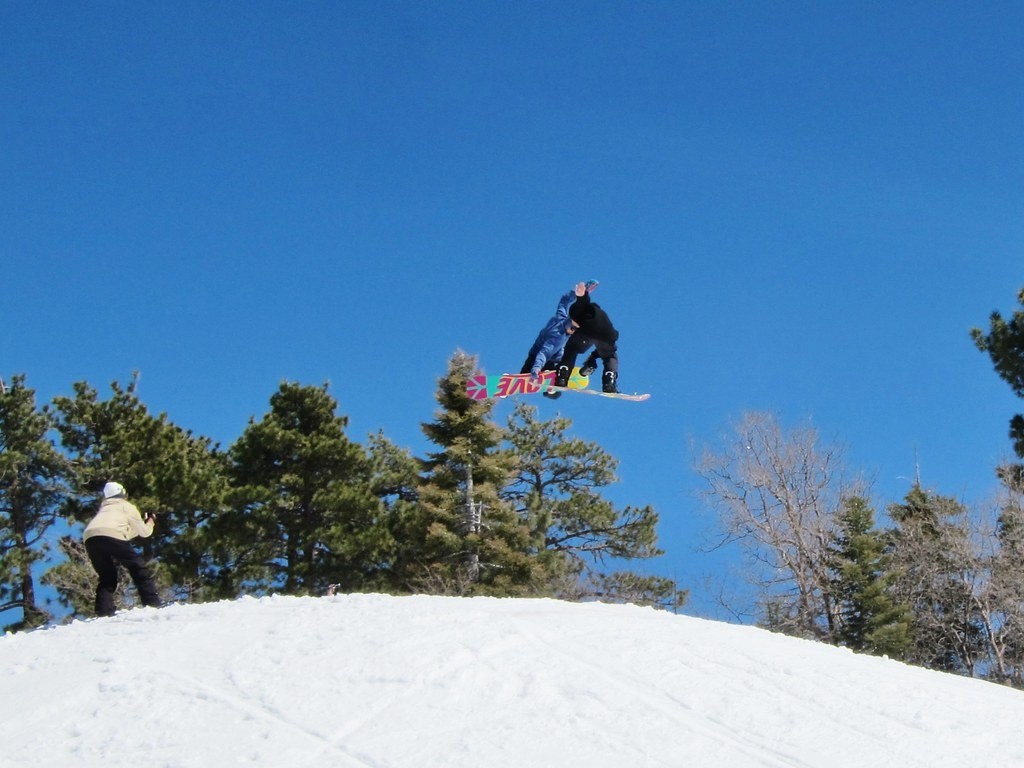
xmin=531 ymin=367 xmax=541 ymax=379
xmin=585 ymin=278 xmax=599 ymax=293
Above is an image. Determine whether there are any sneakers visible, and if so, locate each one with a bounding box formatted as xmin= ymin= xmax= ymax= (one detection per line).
xmin=556 ymin=361 xmax=574 ymax=387
xmin=602 ymin=370 xmax=621 ymax=393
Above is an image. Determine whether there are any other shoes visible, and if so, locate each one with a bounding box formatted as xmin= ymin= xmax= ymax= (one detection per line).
xmin=142 ymin=598 xmax=166 ymax=609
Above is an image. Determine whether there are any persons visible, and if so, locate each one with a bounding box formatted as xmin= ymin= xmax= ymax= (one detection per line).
xmin=521 ymin=280 xmax=620 ymax=400
xmin=82 ymin=482 xmax=160 ymax=618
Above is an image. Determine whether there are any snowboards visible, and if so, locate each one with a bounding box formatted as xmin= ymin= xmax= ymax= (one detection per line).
xmin=464 ymin=362 xmax=651 ymax=402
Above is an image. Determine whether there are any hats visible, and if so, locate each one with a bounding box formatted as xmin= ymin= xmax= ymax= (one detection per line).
xmin=104 ymin=481 xmax=126 ymax=498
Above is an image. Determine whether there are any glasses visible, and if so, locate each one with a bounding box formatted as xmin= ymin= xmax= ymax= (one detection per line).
xmin=568 ymin=319 xmax=579 ymax=331
